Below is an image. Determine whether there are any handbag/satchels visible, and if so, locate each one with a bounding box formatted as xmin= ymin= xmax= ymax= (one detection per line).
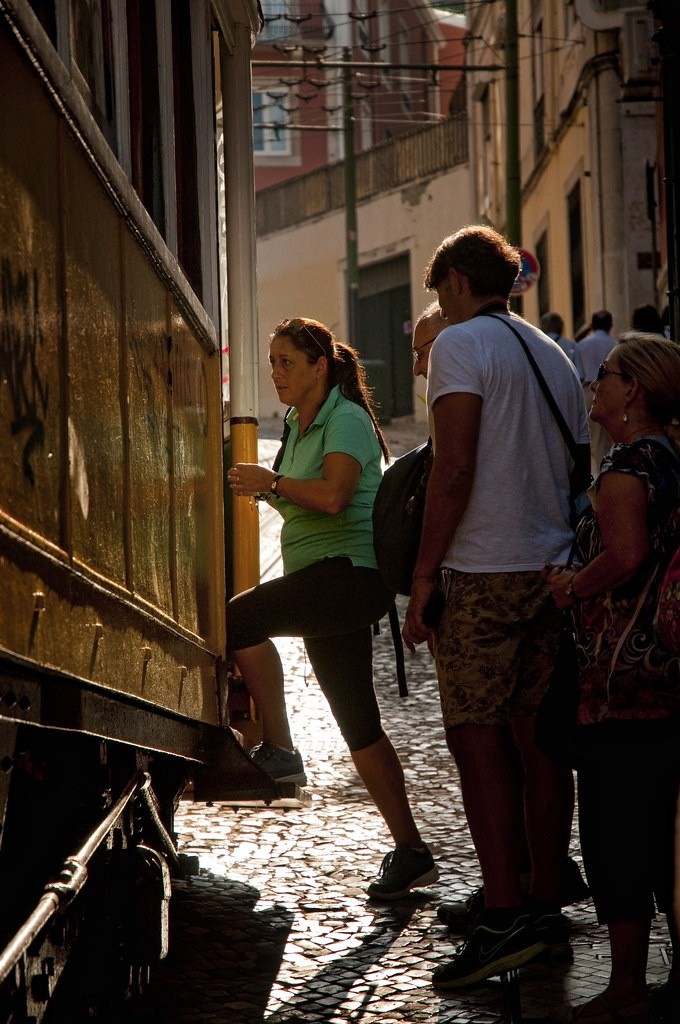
xmin=372 ymin=436 xmax=436 ymax=596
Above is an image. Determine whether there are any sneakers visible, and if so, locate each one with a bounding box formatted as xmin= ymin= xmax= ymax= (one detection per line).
xmin=250 ymin=740 xmax=309 ymax=786
xmin=430 ymin=914 xmax=540 ymax=990
xmin=437 ymin=885 xmax=486 ymax=929
xmin=367 ymin=843 xmax=439 ymax=897
xmin=520 ymin=913 xmax=575 ymax=968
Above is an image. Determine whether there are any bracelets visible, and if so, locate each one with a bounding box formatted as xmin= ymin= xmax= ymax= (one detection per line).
xmin=414 ymin=575 xmax=437 ymax=585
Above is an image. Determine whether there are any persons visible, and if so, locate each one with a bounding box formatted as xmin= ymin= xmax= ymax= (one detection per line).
xmin=538 ymin=312 xmax=586 ymax=383
xmin=401 ymin=226 xmax=592 ymax=988
xmin=544 ymin=336 xmax=680 ymax=1024
xmin=573 ymin=311 xmax=621 ymax=470
xmin=226 ymin=319 xmax=439 ymax=899
xmin=615 ymin=303 xmax=667 ymax=340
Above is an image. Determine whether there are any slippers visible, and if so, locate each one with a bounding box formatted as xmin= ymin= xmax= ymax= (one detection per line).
xmin=552 ymin=995 xmax=590 ymax=1022
xmin=600 ymin=986 xmax=680 ymax=1024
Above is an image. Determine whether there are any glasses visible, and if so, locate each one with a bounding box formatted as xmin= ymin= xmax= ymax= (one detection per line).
xmin=597 ymin=364 xmax=628 ymax=381
xmin=274 ymin=319 xmax=326 ymax=357
xmin=411 ymin=335 xmax=438 ymax=363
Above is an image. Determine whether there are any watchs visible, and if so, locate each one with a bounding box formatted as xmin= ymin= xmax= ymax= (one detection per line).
xmin=270 ymin=475 xmax=284 ymax=499
xmin=565 ymin=575 xmax=583 ymax=601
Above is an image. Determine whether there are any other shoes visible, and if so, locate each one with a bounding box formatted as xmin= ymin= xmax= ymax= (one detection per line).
xmin=555 ymin=860 xmax=589 ymax=905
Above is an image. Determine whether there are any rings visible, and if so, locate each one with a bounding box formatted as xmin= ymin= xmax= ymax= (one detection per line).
xmin=237 ymin=476 xmax=239 ymax=482
